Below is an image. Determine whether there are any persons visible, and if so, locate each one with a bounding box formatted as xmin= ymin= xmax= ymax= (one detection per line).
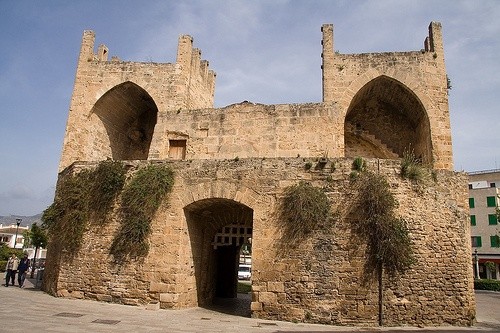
xmin=17 ymin=253 xmax=30 ymax=288
xmin=3 ymin=252 xmax=19 ymax=287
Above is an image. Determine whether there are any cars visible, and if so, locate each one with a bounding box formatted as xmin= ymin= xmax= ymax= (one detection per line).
xmin=31 ymin=258 xmax=39 ymax=269
xmin=39 ymin=258 xmax=46 ymax=268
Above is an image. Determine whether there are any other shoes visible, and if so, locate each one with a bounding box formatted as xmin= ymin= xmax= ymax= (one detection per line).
xmin=19 ymin=284 xmax=24 ymax=288
xmin=4 ymin=284 xmax=7 ymax=287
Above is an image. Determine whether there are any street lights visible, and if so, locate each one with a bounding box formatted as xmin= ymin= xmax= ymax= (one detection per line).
xmin=14 ymin=219 xmax=22 ymax=248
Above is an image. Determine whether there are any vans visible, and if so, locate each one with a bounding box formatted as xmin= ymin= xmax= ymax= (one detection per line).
xmin=238 ymin=264 xmax=251 ymax=279
